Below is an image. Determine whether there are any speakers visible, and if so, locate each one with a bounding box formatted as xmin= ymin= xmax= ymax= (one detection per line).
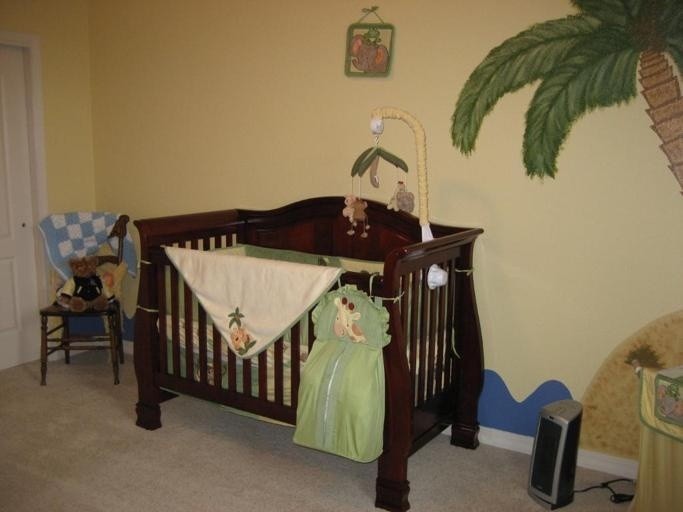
xmin=527 ymin=399 xmax=584 ymax=512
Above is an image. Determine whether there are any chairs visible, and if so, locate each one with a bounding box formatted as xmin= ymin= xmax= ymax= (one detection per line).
xmin=37 ymin=212 xmax=129 ymax=385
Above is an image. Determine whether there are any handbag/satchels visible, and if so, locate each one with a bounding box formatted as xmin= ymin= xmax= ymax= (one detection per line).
xmin=290 ymin=284 xmax=393 ymax=465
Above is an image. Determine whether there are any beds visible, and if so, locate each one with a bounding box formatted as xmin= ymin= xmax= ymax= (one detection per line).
xmin=132 ymin=197 xmax=485 ymax=512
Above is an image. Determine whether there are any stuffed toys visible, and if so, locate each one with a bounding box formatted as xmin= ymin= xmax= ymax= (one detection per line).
xmin=55 ymin=255 xmax=107 ymax=312
xmin=385 ymin=181 xmax=406 ymax=212
xmin=345 ymin=201 xmax=370 ymax=238
xmin=341 ymin=195 xmax=359 ymax=227
xmin=395 ymin=193 xmax=413 ymax=213
